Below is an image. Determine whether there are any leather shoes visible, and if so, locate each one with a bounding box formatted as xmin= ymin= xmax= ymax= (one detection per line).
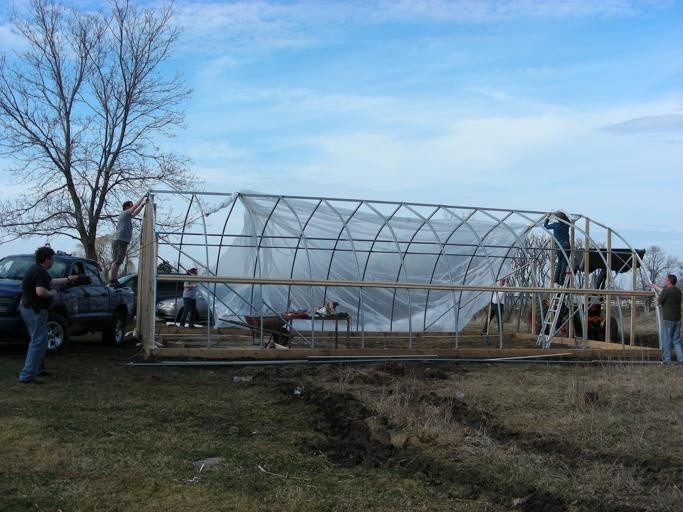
xmin=21 ymin=378 xmax=44 ymax=384
xmin=38 ymin=371 xmax=51 ymax=376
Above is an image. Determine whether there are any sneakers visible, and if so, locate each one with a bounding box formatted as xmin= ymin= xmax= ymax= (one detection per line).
xmin=109 ymin=281 xmax=121 ymax=289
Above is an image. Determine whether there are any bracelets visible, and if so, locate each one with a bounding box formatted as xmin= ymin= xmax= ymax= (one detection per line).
xmin=68 ymin=276 xmax=71 ymax=283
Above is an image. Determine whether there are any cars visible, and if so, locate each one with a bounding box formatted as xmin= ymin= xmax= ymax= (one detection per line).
xmin=157 ymin=285 xmax=215 ymax=325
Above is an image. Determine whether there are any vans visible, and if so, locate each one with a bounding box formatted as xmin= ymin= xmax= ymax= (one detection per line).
xmin=107 ymin=272 xmax=184 ymax=323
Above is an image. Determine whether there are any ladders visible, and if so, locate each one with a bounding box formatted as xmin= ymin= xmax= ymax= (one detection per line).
xmin=534 ymin=255 xmax=575 ymax=349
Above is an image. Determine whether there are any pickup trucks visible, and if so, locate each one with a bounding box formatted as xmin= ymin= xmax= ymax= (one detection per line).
xmin=0 ymin=255 xmax=135 ymax=350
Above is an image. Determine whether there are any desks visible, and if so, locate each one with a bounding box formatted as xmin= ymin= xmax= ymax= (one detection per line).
xmin=282 ymin=314 xmax=351 ymax=349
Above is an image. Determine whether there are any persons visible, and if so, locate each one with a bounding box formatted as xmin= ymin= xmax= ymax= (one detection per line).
xmin=480 ymin=278 xmax=509 ymax=335
xmin=544 ymin=211 xmax=571 ymax=289
xmin=650 ymin=274 xmax=683 ymax=368
xmin=180 ymin=268 xmax=199 ymax=328
xmin=18 ymin=246 xmax=78 ymax=386
xmin=108 ymin=193 xmax=150 ymax=289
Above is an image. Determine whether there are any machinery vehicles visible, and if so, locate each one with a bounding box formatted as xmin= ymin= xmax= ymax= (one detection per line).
xmin=528 ymin=249 xmax=645 ymax=341
